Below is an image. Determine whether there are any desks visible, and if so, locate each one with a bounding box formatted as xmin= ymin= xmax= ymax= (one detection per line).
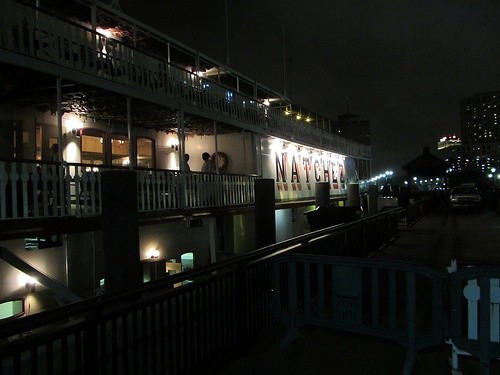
xmin=140 ymin=259 xmax=166 ymax=280
xmin=0 ymin=298 xmax=26 ymax=322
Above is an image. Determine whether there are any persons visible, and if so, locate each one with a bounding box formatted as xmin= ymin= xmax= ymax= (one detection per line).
xmin=182 ymin=153 xmax=191 ymax=172
xmin=48 ymin=143 xmax=67 ymax=208
xmin=201 ymin=152 xmax=211 ymax=173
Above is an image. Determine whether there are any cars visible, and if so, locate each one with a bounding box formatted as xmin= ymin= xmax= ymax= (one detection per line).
xmin=448 ymin=186 xmax=482 ymax=214
